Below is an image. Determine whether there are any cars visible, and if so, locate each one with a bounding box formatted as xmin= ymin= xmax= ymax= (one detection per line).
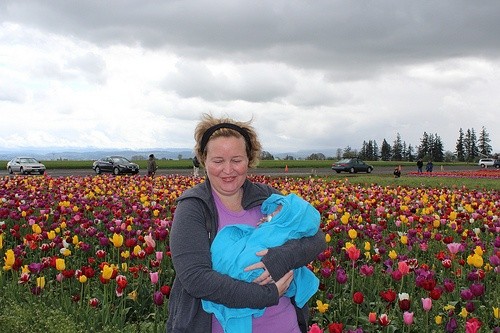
xmin=494 ymin=159 xmax=500 ymax=168
xmin=93 ymin=155 xmax=140 ymax=175
xmin=6 ymin=157 xmax=46 ymax=175
xmin=331 ymin=156 xmax=374 ymax=174
xmin=478 ymin=158 xmax=494 ymax=169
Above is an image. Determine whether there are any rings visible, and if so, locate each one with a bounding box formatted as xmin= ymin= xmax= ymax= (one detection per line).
xmin=270 ymin=275 xmax=273 ymax=280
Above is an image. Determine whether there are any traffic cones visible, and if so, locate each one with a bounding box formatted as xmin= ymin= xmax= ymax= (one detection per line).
xmin=398 ymin=164 xmax=402 ymax=171
xmin=285 ymin=165 xmax=289 ymax=172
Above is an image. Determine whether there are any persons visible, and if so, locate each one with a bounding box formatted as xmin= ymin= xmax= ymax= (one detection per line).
xmin=147 ymin=154 xmax=157 ymax=178
xmin=201 ymin=192 xmax=321 ymax=333
xmin=494 ymin=159 xmax=500 ymax=168
xmin=192 ymin=156 xmax=200 ymax=177
xmin=394 ymin=157 xmax=433 ymax=177
xmin=165 ymin=112 xmax=328 ymax=333
